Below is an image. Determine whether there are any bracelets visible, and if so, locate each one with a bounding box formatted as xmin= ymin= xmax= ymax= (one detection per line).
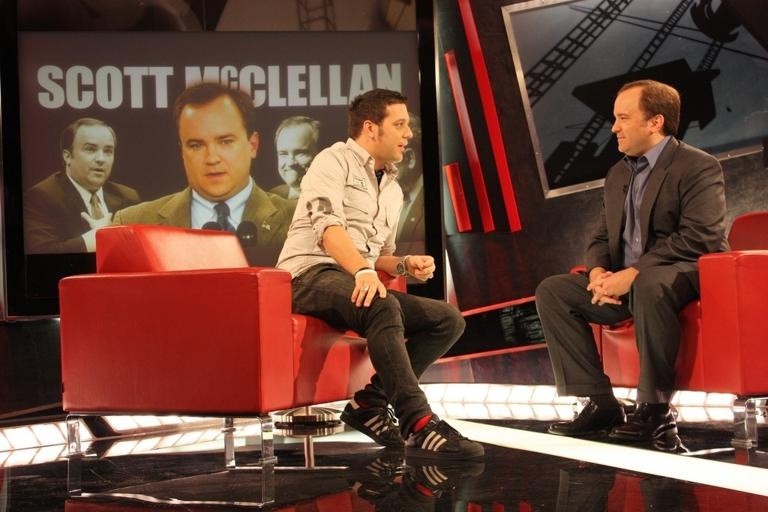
xmin=355 ymin=267 xmax=376 ymax=277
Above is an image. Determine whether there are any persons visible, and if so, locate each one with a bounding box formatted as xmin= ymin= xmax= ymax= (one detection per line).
xmin=271 ymin=115 xmax=316 ymax=204
xmin=381 ymin=113 xmax=425 ymax=291
xmin=278 ymin=88 xmax=485 ymax=461
xmin=344 ymin=444 xmax=472 ymax=512
xmin=534 ymin=80 xmax=727 ymax=453
xmin=23 ymin=117 xmax=141 ymax=257
xmin=112 ymin=82 xmax=298 ymax=271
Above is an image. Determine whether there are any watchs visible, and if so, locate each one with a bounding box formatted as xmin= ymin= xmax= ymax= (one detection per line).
xmin=397 ymin=254 xmax=411 ymax=277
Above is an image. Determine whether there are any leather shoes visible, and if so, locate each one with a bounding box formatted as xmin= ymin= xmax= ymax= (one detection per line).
xmin=548 ymin=401 xmax=627 ymax=436
xmin=609 ymin=403 xmax=678 ymax=442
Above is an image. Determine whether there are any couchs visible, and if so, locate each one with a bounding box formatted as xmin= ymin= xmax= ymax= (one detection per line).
xmin=569 ymin=210 xmax=768 ymax=451
xmin=58 ymin=224 xmax=408 ymax=508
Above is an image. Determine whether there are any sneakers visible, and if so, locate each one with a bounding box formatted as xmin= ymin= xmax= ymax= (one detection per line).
xmin=341 ymin=399 xmax=405 ymax=447
xmin=347 ymin=448 xmax=405 ymax=499
xmin=413 ymin=460 xmax=484 ymax=497
xmin=406 ymin=414 xmax=484 ymax=459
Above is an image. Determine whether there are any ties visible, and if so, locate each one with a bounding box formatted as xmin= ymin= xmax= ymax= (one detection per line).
xmin=214 ymin=203 xmax=230 ymax=230
xmin=91 ymin=195 xmax=104 ymax=220
